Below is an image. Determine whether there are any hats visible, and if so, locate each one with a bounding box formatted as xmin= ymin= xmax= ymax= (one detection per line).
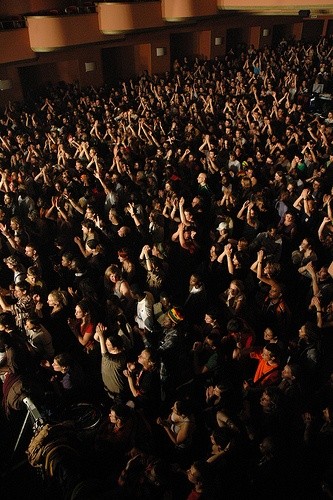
xmin=154 ymin=242 xmax=168 ymax=258
xmin=167 ymin=306 xmax=184 ymax=325
xmin=216 ymin=222 xmax=229 ymax=230
xmin=3 ymin=255 xmax=17 ymax=266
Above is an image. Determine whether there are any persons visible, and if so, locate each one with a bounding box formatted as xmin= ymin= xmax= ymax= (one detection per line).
xmin=0 ymin=33 xmax=333 ymax=500
xmin=185 ymin=460 xmax=218 ymax=500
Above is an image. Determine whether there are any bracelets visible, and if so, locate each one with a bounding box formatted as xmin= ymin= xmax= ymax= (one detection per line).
xmin=258 ymin=261 xmax=263 ymax=264
xmin=6 ymin=234 xmax=12 ymax=239
xmin=146 ymin=258 xmax=151 ymax=260
xmin=303 ymin=198 xmax=307 ymax=200
xmin=57 ymin=207 xmax=61 ymax=210
xmin=226 ymin=197 xmax=229 ymax=199
xmin=67 ymin=198 xmax=74 ymax=203
xmin=131 ymin=212 xmax=136 ymax=218
